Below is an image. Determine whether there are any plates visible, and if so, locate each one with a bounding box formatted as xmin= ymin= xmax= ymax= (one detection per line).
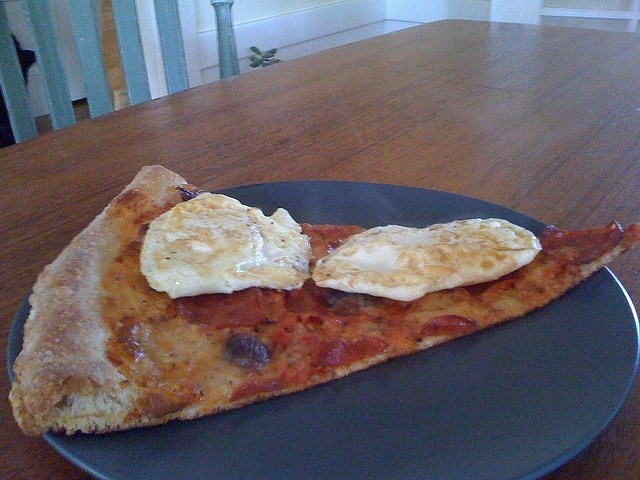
xmin=6 ymin=180 xmax=640 ymax=476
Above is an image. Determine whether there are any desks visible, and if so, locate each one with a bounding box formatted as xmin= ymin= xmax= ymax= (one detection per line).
xmin=0 ymin=19 xmax=640 ymax=480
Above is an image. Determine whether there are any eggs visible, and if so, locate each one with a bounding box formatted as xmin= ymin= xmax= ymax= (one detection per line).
xmin=139 ymin=191 xmax=312 ymax=300
xmin=313 ymin=218 xmax=543 ymax=302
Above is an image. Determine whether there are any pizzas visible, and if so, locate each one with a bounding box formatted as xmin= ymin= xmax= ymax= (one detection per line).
xmin=9 ymin=165 xmax=639 ymax=439
